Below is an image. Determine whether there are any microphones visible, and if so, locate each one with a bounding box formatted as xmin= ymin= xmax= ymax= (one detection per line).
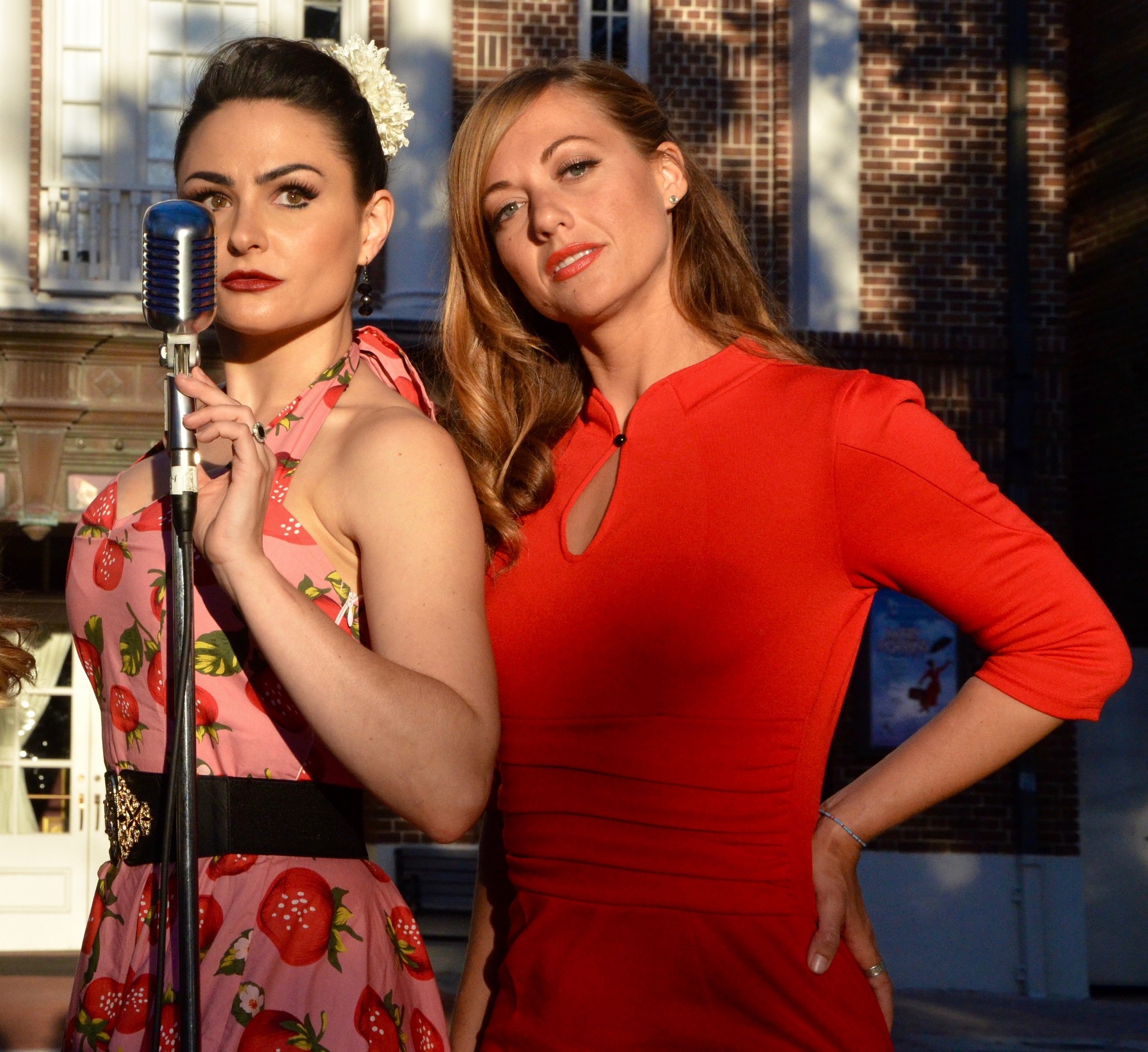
xmin=139 ymin=198 xmax=218 ymax=540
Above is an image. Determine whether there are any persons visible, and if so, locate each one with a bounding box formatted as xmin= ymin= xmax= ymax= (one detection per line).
xmin=437 ymin=61 xmax=1133 ymax=1052
xmin=62 ymin=37 xmax=498 ymax=1052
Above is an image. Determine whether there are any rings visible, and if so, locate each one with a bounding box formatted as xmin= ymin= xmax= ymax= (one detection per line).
xmin=252 ymin=422 xmax=267 ymax=443
xmin=865 ymin=960 xmax=886 ymax=978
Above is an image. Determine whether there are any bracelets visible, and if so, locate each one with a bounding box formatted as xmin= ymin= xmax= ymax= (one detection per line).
xmin=819 ymin=808 xmax=867 ymax=849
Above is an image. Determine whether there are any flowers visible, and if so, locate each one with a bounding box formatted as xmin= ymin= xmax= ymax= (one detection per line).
xmin=320 ymin=33 xmax=416 ymax=161
